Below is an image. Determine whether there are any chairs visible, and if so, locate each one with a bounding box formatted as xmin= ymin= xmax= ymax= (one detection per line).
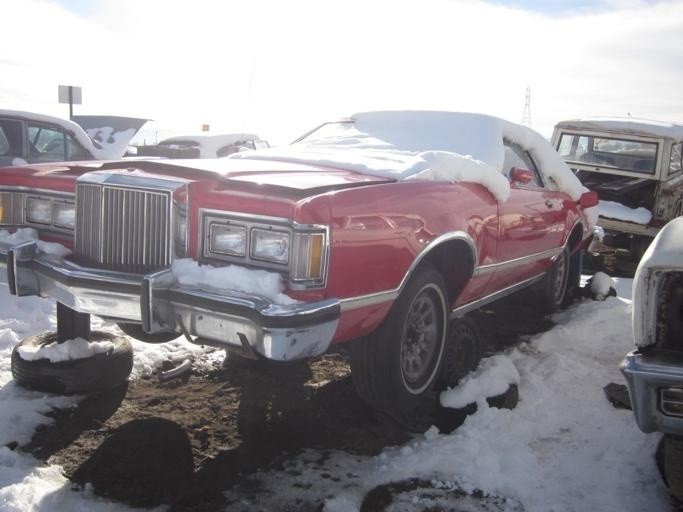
xmin=579 ymin=151 xmax=654 ymax=173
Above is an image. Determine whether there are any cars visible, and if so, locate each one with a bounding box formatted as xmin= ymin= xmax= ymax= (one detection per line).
xmin=160 ymin=132 xmax=272 ymax=161
xmin=0 ymin=108 xmax=150 ymax=168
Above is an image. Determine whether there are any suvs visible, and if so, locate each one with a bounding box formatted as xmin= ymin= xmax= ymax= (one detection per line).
xmin=602 ymin=216 xmax=682 ymax=438
xmin=550 ymin=120 xmax=682 ymax=259
xmin=4 ymin=110 xmax=599 ymax=422
